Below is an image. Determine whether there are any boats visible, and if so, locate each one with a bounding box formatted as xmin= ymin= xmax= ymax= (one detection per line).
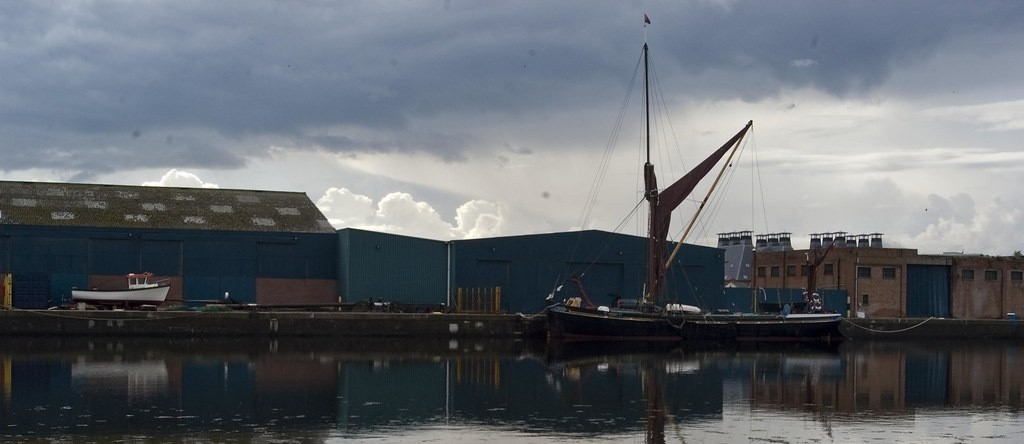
xmin=70 ymin=271 xmax=172 ymax=308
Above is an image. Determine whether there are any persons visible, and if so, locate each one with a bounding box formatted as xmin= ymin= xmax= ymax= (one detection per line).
xmin=617 ymin=297 xmax=621 ymax=310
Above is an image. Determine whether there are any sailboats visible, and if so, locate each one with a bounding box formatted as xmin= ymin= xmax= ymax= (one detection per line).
xmin=546 ymin=44 xmax=842 ymax=340
